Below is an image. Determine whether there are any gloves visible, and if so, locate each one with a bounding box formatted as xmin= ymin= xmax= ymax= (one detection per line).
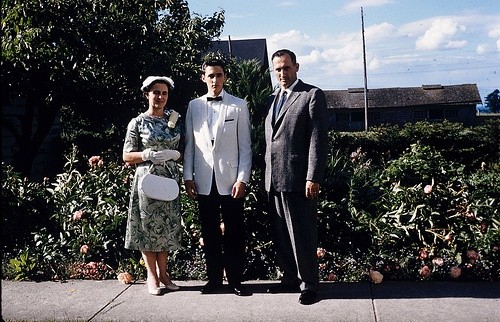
xmin=141 ymin=148 xmax=181 ymax=165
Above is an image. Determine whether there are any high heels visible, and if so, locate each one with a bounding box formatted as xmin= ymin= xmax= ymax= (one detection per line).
xmin=147 ymin=278 xmax=161 ymax=295
xmin=159 ymin=273 xmax=179 ymax=290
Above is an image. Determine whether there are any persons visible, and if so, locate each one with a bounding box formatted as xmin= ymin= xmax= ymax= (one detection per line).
xmin=123 ymin=76 xmax=183 ymax=295
xmin=264 ymin=49 xmax=328 ymax=305
xmin=186 ymin=58 xmax=253 ymax=296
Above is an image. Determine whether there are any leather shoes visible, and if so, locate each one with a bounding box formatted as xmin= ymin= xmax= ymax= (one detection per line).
xmin=233 ymin=283 xmax=252 ymax=296
xmin=299 ymin=282 xmax=323 ymax=305
xmin=200 ymin=278 xmax=223 ymax=293
xmin=267 ymin=274 xmax=298 ymax=293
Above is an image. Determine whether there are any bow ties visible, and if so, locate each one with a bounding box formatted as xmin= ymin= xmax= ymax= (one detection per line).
xmin=207 ymin=96 xmax=223 ymax=102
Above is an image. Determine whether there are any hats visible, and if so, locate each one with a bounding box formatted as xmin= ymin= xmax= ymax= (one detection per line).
xmin=140 ymin=75 xmax=174 ymax=93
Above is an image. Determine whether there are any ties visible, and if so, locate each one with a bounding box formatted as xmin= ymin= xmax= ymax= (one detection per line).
xmin=275 ymin=90 xmax=287 ymax=123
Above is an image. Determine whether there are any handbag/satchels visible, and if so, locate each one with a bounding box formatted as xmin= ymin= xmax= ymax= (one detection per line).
xmin=140 ymin=162 xmax=180 ymax=201
